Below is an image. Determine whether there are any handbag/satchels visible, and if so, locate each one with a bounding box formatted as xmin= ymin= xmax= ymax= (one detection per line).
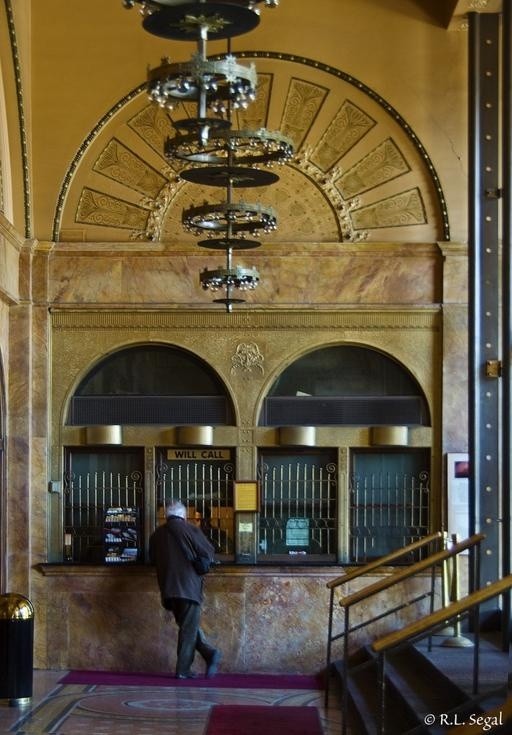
xmin=193 ymin=556 xmax=209 ymax=575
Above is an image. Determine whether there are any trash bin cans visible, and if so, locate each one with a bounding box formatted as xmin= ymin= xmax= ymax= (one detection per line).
xmin=0 ymin=592 xmax=34 ymax=707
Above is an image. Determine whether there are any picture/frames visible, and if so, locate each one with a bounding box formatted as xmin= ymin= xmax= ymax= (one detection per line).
xmin=233 ymin=480 xmax=260 ymax=513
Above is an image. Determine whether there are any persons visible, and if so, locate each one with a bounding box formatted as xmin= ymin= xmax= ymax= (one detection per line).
xmin=148 ymin=500 xmax=224 ymax=679
xmin=192 ymin=503 xmax=235 ymax=553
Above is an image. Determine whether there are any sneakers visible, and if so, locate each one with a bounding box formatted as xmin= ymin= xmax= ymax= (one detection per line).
xmin=177 ymin=672 xmax=198 ymax=679
xmin=205 ymin=648 xmax=221 ymax=679
